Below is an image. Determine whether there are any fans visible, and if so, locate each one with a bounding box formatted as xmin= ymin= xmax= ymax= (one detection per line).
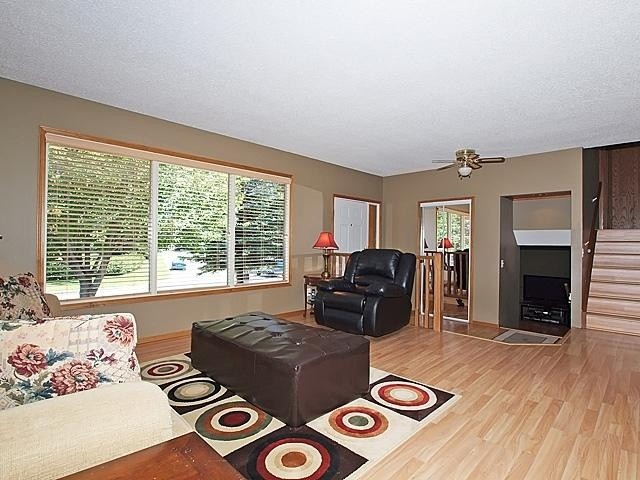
xmin=432 ymin=149 xmax=505 ymax=171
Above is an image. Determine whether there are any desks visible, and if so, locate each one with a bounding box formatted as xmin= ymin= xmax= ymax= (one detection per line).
xmin=302 ymin=274 xmax=341 ymax=317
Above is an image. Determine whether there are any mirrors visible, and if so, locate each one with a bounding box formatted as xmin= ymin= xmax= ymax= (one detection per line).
xmin=417 ymin=197 xmax=472 ymax=323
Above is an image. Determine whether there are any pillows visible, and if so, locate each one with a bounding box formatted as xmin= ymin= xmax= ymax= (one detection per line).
xmin=0 ymin=273 xmax=141 ymax=411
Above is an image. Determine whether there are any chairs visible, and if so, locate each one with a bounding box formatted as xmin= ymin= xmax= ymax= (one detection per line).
xmin=0 ymin=273 xmax=174 ymax=480
xmin=455 ymin=249 xmax=470 ymax=291
xmin=313 ymin=250 xmax=419 ymax=337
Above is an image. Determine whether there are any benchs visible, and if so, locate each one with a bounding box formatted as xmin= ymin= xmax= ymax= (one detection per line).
xmin=192 ymin=311 xmax=370 ymax=427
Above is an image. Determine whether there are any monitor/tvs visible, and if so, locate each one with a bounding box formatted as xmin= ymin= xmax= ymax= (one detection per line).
xmin=522 ymin=274 xmax=570 ymax=305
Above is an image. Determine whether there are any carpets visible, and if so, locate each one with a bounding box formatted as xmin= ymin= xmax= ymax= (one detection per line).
xmin=139 ymin=352 xmax=462 ymax=480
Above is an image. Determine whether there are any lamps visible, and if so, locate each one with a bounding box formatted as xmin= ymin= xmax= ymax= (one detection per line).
xmin=437 ymin=238 xmax=454 ymax=252
xmin=458 ymin=167 xmax=472 ymax=178
xmin=313 ymin=231 xmax=339 ymax=278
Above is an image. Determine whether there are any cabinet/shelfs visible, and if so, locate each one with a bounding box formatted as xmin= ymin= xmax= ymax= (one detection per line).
xmin=520 ymin=302 xmax=569 ymax=326
